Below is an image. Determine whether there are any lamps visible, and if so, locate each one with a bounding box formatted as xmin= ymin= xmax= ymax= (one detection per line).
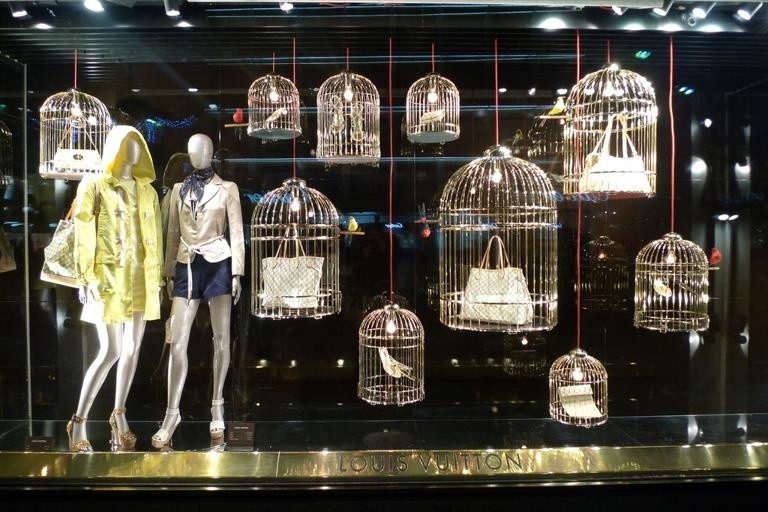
xmin=7 ymin=1 xmax=56 ymax=30
xmin=163 ymin=0 xmax=181 ymax=17
xmin=611 ymin=0 xmax=764 ymax=21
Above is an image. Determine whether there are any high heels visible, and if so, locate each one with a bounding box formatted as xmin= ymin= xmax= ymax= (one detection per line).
xmin=110 ymin=407 xmax=138 ymax=449
xmin=67 ymin=413 xmax=93 ymax=454
xmin=152 ymin=409 xmax=182 ymax=448
xmin=210 ymin=399 xmax=225 ymax=440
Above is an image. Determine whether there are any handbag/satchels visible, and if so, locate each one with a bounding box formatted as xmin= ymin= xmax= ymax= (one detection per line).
xmin=54 ymin=117 xmax=100 ymax=172
xmin=460 ymin=235 xmax=534 ymax=325
xmin=579 ymin=115 xmax=652 ymax=192
xmin=260 ymin=224 xmax=324 ymax=309
xmin=40 ymin=220 xmax=79 ymax=289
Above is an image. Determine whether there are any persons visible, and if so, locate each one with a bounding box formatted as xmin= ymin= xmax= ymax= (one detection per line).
xmin=65 ymin=124 xmax=166 ymax=452
xmin=150 ymin=133 xmax=245 ymax=449
